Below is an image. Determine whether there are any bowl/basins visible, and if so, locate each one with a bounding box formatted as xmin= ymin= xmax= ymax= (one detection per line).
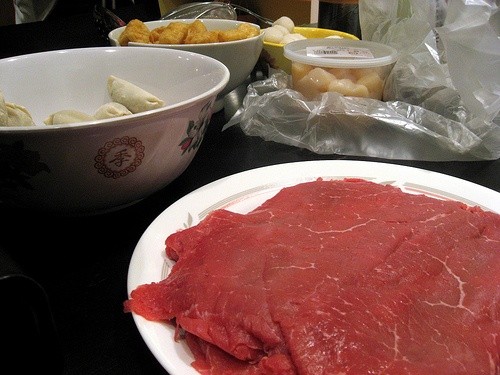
xmin=108 ymin=19 xmax=266 ymax=114
xmin=0 ymin=47 xmax=231 ymax=218
xmin=261 ymin=27 xmax=359 ymax=75
xmin=284 ymin=38 xmax=399 ymax=102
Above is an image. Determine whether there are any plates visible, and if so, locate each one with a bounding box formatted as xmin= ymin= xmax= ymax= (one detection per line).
xmin=127 ymin=160 xmax=500 ymax=375
